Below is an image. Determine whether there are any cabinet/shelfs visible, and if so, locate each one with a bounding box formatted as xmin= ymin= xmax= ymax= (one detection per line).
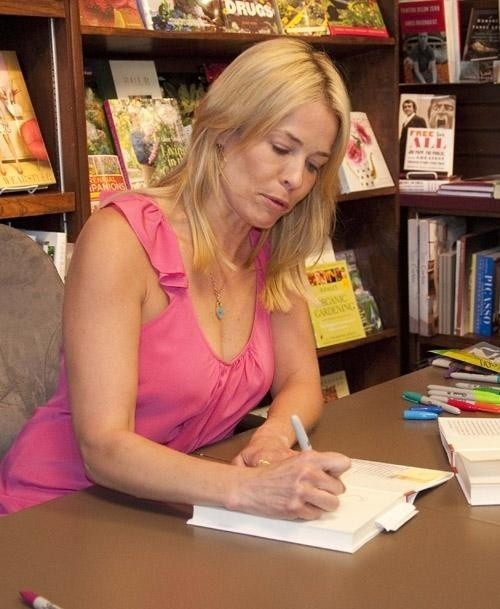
xmin=399 ymin=0 xmax=500 ymax=369
xmin=2 ymin=0 xmax=403 ymax=465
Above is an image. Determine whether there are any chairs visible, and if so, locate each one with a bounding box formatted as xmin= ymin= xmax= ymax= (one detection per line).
xmin=1 ymin=224 xmax=66 ymax=458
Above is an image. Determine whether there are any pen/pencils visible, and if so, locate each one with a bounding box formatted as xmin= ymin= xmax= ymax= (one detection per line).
xmin=402 ymin=390 xmax=461 ymax=416
xmin=450 ymin=372 xmax=499 ymax=383
xmin=290 ymin=413 xmax=312 ymax=455
xmin=429 ymin=394 xmax=480 ymax=415
xmin=448 ymin=397 xmax=499 ymax=413
xmin=456 ymin=383 xmax=500 ymax=392
xmin=427 ymin=389 xmax=498 ymax=403
xmin=427 ymin=383 xmax=500 ymax=401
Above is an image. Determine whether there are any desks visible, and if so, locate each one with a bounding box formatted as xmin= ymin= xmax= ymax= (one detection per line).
xmin=0 ymin=362 xmax=499 ymax=609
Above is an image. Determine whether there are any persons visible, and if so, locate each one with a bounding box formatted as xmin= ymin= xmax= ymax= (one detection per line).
xmin=129 ymin=112 xmax=158 ymax=165
xmin=411 ymin=32 xmax=437 ymax=83
xmin=0 ymin=38 xmax=352 ymax=519
xmin=428 ymin=96 xmax=455 ymax=129
xmin=399 ymin=99 xmax=427 ymax=171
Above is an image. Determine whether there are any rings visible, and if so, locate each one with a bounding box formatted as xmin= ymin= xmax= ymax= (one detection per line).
xmin=256 ymin=459 xmax=270 ymax=468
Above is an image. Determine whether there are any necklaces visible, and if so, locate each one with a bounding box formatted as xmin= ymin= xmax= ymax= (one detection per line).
xmin=194 ymin=202 xmax=244 ymax=319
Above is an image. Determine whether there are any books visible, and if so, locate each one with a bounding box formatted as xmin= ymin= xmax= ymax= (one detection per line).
xmin=78 ymin=0 xmax=145 ymax=29
xmin=0 ymin=50 xmax=57 ymax=194
xmin=137 ymin=0 xmax=224 ymax=34
xmin=221 ymin=0 xmax=281 ymax=35
xmin=337 ymin=168 xmax=346 ymax=195
xmin=19 ymin=228 xmax=66 ymax=285
xmin=88 ymin=156 xmax=127 ymax=215
xmin=324 ymin=0 xmax=389 ymax=38
xmin=408 ymin=212 xmax=500 ymax=335
xmin=454 ymin=0 xmax=500 ymax=83
xmin=109 ymin=61 xmax=162 ymax=98
xmin=84 ymin=58 xmax=118 ymax=155
xmin=273 ymin=0 xmax=330 ymax=36
xmin=437 ymin=418 xmax=500 ymax=507
xmin=317 ymin=370 xmax=350 ymax=402
xmin=355 ymin=291 xmax=372 ymax=301
xmin=199 ymin=63 xmax=231 ymax=92
xmin=104 ymin=100 xmax=190 ymax=190
xmin=357 ymin=297 xmax=384 ymax=334
xmin=158 ymin=72 xmax=208 ymax=127
xmin=436 ymin=190 xmax=493 ymax=198
xmin=398 ymin=0 xmax=458 ymax=84
xmin=341 ymin=112 xmax=395 ymax=193
xmin=66 ymin=243 xmax=73 ymax=276
xmin=304 ymin=237 xmax=335 ymax=268
xmin=243 ymin=404 xmax=269 ymax=428
xmin=187 ymin=451 xmax=454 ymax=554
xmin=429 ymin=341 xmax=500 ymax=373
xmin=306 ymin=260 xmax=367 ymax=348
xmin=439 ymin=174 xmax=500 ymax=192
xmin=398 ymin=93 xmax=457 ymax=179
xmin=335 ymin=251 xmax=363 ymax=293
